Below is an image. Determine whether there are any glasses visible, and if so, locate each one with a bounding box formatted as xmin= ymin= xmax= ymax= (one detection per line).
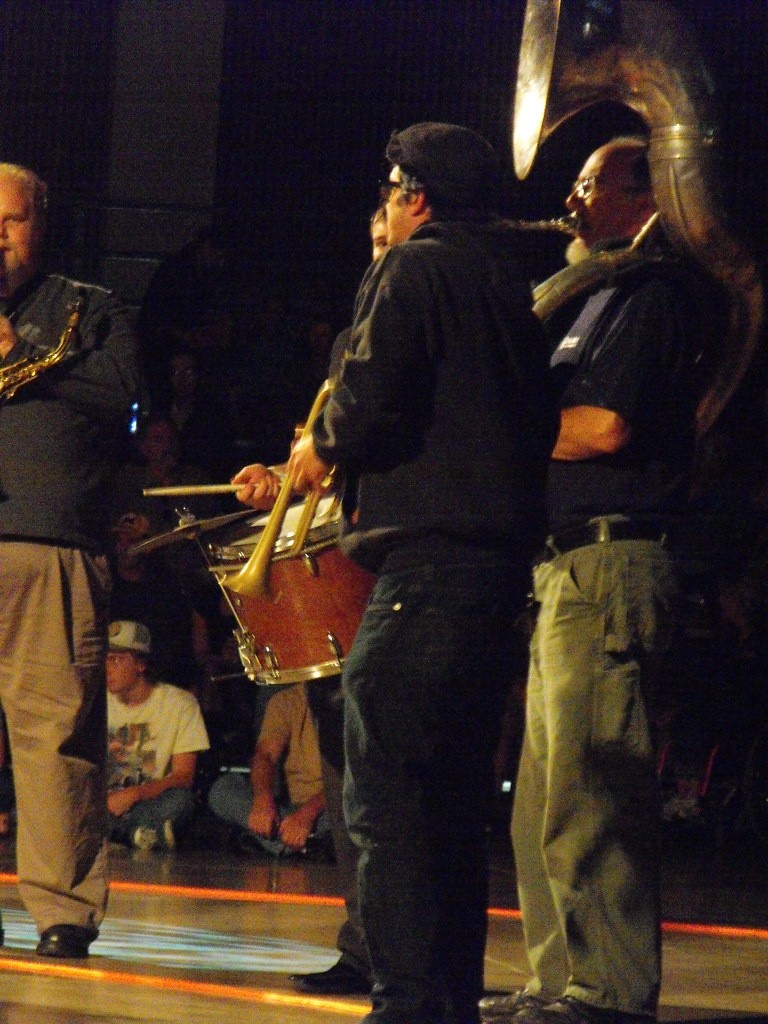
xmin=574 ymin=177 xmax=628 ymax=198
xmin=376 ymin=178 xmax=415 ymax=199
xmin=105 ymin=656 xmax=126 ymax=667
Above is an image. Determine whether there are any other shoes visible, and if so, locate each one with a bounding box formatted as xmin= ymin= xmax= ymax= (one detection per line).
xmin=478 ymin=988 xmax=559 ymax=1023
xmin=509 ymin=995 xmax=657 ymax=1024
xmin=132 ymin=820 xmax=176 ymax=855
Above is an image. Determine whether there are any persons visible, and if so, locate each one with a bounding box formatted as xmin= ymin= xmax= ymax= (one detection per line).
xmin=0 ymin=120 xmax=768 ymax=1024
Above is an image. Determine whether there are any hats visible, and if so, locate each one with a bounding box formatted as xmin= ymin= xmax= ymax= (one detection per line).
xmin=387 ymin=122 xmax=501 ymax=202
xmin=108 ymin=619 xmax=155 ymax=654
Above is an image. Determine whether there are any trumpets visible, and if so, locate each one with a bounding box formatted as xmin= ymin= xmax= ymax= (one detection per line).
xmin=218 ymin=353 xmax=335 ymax=603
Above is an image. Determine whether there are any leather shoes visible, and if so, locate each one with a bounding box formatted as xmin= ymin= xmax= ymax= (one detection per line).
xmin=287 ymin=963 xmax=375 ymax=995
xmin=35 ymin=923 xmax=94 ymax=958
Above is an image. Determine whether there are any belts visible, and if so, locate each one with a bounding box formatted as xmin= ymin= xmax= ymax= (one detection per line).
xmin=539 ymin=519 xmax=679 ymax=561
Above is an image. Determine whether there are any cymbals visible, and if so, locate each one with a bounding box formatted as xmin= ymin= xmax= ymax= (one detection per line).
xmin=126 ymin=509 xmax=259 ymax=557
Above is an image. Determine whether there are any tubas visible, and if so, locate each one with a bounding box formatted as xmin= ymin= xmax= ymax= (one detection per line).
xmin=486 ymin=0 xmax=768 ymax=449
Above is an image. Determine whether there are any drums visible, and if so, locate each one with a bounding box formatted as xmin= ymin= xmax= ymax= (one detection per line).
xmin=210 ymin=491 xmax=381 ymax=686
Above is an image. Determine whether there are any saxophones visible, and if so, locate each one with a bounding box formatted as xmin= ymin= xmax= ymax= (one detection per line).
xmin=0 ymin=296 xmax=84 ymax=405
